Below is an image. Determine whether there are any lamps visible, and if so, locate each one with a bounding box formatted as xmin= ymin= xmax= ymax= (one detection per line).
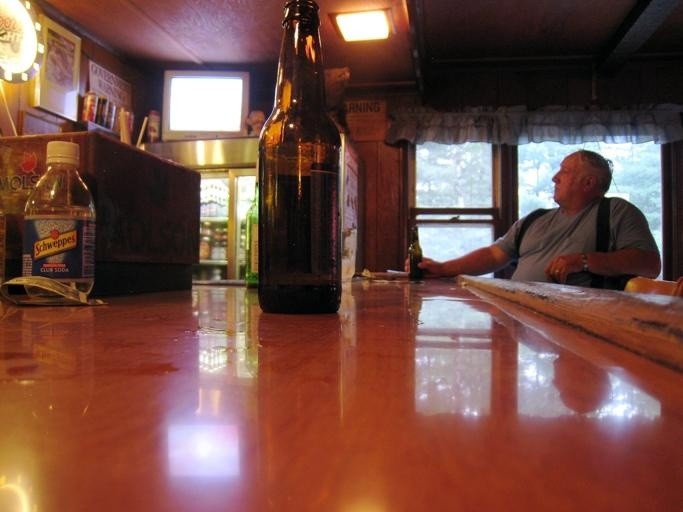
xmin=324 ymin=5 xmax=396 ymax=49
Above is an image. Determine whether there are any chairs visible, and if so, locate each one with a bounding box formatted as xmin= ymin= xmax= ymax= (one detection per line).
xmin=618 ymin=275 xmax=682 ymax=299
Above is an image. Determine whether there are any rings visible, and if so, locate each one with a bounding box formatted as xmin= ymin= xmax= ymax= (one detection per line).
xmin=555 ymin=269 xmax=560 ymax=274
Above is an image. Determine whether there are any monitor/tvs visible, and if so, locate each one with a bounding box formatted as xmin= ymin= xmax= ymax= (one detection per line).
xmin=161 ymin=71 xmax=250 ymax=142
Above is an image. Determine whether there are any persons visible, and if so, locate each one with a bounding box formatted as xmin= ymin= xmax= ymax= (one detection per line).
xmin=404 ymin=151 xmax=661 ymax=288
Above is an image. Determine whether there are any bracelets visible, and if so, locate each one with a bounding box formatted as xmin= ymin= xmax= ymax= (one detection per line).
xmin=583 ymin=252 xmax=589 ymax=270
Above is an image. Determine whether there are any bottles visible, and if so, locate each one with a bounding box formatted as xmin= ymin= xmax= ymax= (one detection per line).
xmin=406 ymin=224 xmax=425 ymax=282
xmin=80 ymin=91 xmax=161 ymax=144
xmin=20 ymin=139 xmax=98 ymax=300
xmin=198 ymin=221 xmax=245 ymax=263
xmin=244 ymin=0 xmax=344 ymax=316
